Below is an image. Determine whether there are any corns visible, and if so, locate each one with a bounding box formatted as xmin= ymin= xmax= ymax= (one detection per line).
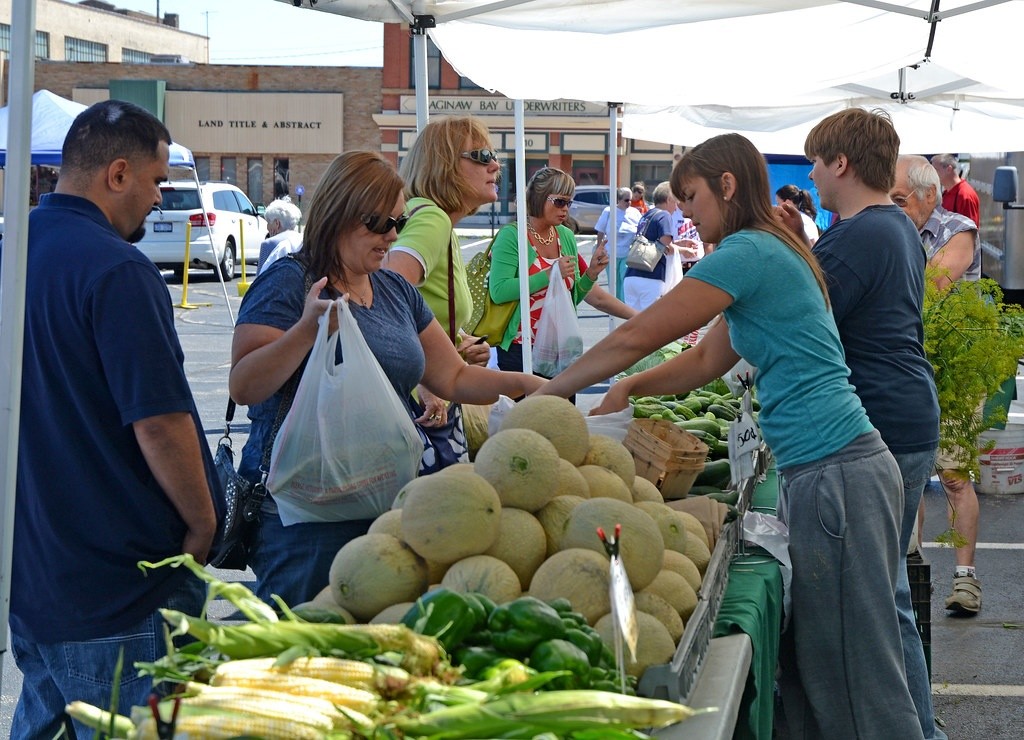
xmin=122 ymin=619 xmax=696 ymax=740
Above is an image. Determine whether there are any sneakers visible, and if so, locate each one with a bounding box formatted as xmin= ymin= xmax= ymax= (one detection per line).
xmin=944 ymin=571 xmax=981 ymax=612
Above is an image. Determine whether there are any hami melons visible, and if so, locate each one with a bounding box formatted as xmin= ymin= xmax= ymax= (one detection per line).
xmin=364 ymin=603 xmax=416 ymax=623
xmin=290 ymin=395 xmax=715 ymax=605
xmin=626 ymin=590 xmax=683 ymax=645
xmin=328 ymin=534 xmax=430 ymax=620
xmin=589 ymin=608 xmax=678 ymax=675
xmin=289 ymin=600 xmax=357 ymax=624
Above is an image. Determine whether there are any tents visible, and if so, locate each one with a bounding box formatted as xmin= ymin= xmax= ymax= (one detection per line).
xmin=275 ymin=0 xmax=1024 ymax=380
xmin=0 ymin=88 xmax=235 ymax=329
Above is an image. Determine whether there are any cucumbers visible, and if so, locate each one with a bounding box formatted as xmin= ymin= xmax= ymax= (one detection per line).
xmin=624 ymin=387 xmax=762 ymax=499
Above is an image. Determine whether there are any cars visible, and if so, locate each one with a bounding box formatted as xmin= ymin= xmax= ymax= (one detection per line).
xmin=129 ymin=177 xmax=269 ymax=281
xmin=561 ymin=184 xmax=655 ymax=235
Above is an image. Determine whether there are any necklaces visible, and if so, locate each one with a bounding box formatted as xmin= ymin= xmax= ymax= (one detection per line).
xmin=527 ymin=221 xmax=554 ymax=246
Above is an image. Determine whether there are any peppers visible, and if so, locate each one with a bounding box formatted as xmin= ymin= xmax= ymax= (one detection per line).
xmin=278 ymin=588 xmax=638 ymax=697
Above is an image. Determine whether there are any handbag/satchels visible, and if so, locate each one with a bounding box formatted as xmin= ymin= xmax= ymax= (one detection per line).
xmin=406 ymin=393 xmax=469 ymax=478
xmin=461 ymin=223 xmax=519 ymax=347
xmin=626 ymin=210 xmax=670 ymax=272
xmin=663 ymin=244 xmax=683 ymax=294
xmin=265 ymin=297 xmax=424 ymax=526
xmin=205 ymin=442 xmax=261 ymax=570
xmin=592 ymin=240 xmax=599 ymax=256
xmin=532 ymin=261 xmax=583 ymax=378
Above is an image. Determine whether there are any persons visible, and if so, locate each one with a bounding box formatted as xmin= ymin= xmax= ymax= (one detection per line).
xmin=489 ymin=167 xmax=640 ymax=405
xmin=524 ymin=135 xmax=925 ymax=740
xmin=595 ymin=181 xmax=714 ymax=313
xmin=256 ymin=194 xmax=304 ymax=278
xmin=382 ymin=116 xmax=491 ymax=477
xmin=767 ymin=107 xmax=981 ymax=740
xmin=0 ymin=99 xmax=227 ymax=740
xmin=227 ymin=149 xmax=549 ymax=616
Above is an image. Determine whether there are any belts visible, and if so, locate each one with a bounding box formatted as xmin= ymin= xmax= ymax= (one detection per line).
xmin=681 ymin=262 xmax=696 ymax=268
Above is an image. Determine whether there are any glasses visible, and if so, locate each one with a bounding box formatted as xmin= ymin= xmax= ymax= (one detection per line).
xmin=459 ymin=148 xmax=498 ymax=165
xmin=892 ymin=186 xmax=921 ymax=207
xmin=357 ymin=212 xmax=409 ymax=235
xmin=620 ymin=198 xmax=632 ymax=202
xmin=547 ymin=196 xmax=573 ymax=209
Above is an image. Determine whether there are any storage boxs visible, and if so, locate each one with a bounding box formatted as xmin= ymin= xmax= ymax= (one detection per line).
xmin=624 ymin=418 xmax=708 ymax=501
xmin=969 ymin=358 xmax=1024 ymax=494
xmin=906 ymin=553 xmax=932 ymax=695
